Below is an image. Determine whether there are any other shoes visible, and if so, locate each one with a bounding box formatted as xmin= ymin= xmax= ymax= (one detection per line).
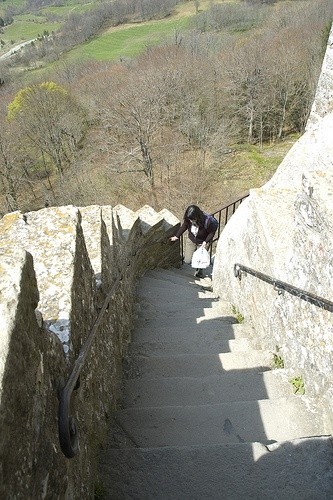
xmin=196 ymin=272 xmax=203 ymax=278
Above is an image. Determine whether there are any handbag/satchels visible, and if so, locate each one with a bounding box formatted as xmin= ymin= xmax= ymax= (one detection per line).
xmin=191 ymin=246 xmax=211 ymax=269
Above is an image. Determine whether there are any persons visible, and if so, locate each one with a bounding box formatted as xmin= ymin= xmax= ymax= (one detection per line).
xmin=169 ymin=205 xmax=218 ymax=278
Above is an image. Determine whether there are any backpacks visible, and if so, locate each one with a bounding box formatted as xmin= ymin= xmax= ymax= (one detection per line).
xmin=203 ymin=214 xmax=218 ymax=229
